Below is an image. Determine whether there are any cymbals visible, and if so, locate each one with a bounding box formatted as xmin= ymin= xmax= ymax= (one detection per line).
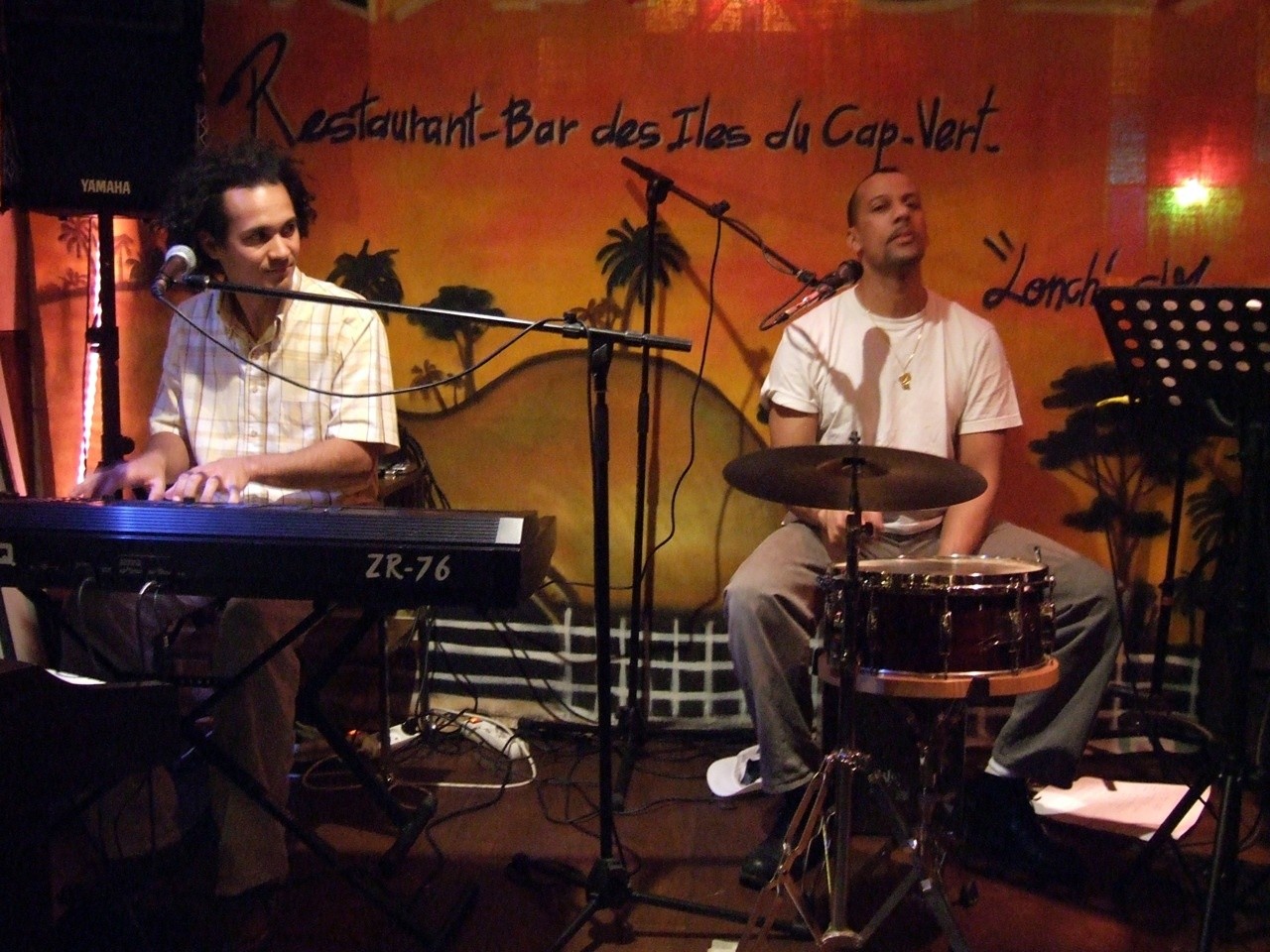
xmin=723 ymin=433 xmax=989 ymax=513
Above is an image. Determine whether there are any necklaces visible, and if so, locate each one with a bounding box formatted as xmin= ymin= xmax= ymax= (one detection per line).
xmin=855 ymin=287 xmax=929 ymax=387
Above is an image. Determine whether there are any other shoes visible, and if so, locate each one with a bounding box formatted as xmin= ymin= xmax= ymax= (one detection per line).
xmin=739 ymin=774 xmax=858 ymax=892
xmin=956 ymin=769 xmax=1086 ymax=890
xmin=103 ymin=862 xmax=181 ymax=919
xmin=180 ymin=898 xmax=271 ymax=952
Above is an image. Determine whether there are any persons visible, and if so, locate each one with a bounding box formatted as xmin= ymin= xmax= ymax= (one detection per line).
xmin=723 ymin=167 xmax=1122 ymax=888
xmin=3 ymin=154 xmax=400 ymax=925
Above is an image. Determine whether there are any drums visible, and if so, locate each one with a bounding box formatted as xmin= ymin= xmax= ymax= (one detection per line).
xmin=816 ymin=555 xmax=1058 ymax=698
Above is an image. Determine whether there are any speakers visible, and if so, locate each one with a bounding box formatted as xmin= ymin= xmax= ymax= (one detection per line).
xmin=0 ymin=0 xmax=202 ymax=220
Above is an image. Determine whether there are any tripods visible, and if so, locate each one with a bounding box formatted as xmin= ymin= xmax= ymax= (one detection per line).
xmin=1093 ymin=285 xmax=1270 ymax=952
xmin=178 ymin=160 xmax=968 ymax=952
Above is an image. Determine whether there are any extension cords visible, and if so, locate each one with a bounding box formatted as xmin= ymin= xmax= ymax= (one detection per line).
xmin=390 ymin=715 xmax=436 ymax=751
xmin=461 ymin=718 xmax=531 ymax=761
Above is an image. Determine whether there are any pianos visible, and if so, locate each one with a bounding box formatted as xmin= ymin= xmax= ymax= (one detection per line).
xmin=1 ymin=498 xmax=557 ymax=901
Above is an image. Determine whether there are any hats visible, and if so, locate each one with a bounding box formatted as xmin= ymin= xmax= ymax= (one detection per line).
xmin=705 ymin=744 xmax=763 ymax=798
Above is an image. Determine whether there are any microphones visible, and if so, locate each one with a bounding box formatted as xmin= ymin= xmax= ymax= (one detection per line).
xmin=775 ymin=260 xmax=864 ymax=325
xmin=149 ymin=243 xmax=196 ymax=299
